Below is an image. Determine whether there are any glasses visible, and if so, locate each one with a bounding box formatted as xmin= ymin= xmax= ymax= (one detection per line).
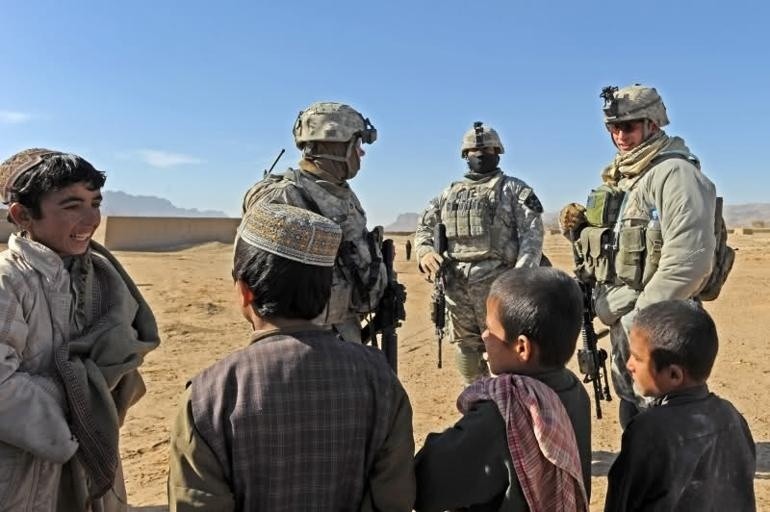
xmin=605 ymin=121 xmax=637 ymax=135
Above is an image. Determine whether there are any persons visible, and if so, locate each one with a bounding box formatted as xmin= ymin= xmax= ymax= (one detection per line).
xmin=167 ymin=199 xmax=416 ymax=512
xmin=412 ymin=266 xmax=591 ymax=511
xmin=412 ymin=116 xmax=553 ymax=391
xmin=0 ymin=148 xmax=160 ymax=511
xmin=404 ymin=238 xmax=415 ymax=262
xmin=556 ymin=80 xmax=717 ymax=431
xmin=601 ymin=297 xmax=757 ymax=512
xmin=234 ymin=99 xmax=389 ymax=352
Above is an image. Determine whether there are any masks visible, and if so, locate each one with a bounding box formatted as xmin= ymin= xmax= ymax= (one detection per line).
xmin=467 ymin=152 xmax=500 ymax=174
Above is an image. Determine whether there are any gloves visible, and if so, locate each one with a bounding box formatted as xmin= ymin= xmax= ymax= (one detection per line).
xmin=420 ymin=252 xmax=443 ymax=276
xmin=559 ymin=203 xmax=586 ymax=231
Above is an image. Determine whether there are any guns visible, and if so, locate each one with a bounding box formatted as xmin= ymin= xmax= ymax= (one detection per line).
xmin=423 ymin=220 xmax=452 ymax=370
xmin=570 ymin=265 xmax=612 ymax=421
xmin=359 ymin=238 xmax=410 ymax=377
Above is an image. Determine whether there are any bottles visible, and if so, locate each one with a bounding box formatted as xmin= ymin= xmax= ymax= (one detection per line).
xmin=647 ymin=212 xmax=661 ymax=232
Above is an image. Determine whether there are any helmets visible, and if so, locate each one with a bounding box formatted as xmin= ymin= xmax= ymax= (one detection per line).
xmin=293 ymin=102 xmax=377 ymax=179
xmin=461 ymin=121 xmax=504 ymax=158
xmin=600 ymin=84 xmax=670 ymax=151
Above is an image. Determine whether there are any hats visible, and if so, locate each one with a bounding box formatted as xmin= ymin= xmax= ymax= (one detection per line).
xmin=0 ymin=147 xmax=58 ymax=206
xmin=237 ymin=203 xmax=343 ymax=267
